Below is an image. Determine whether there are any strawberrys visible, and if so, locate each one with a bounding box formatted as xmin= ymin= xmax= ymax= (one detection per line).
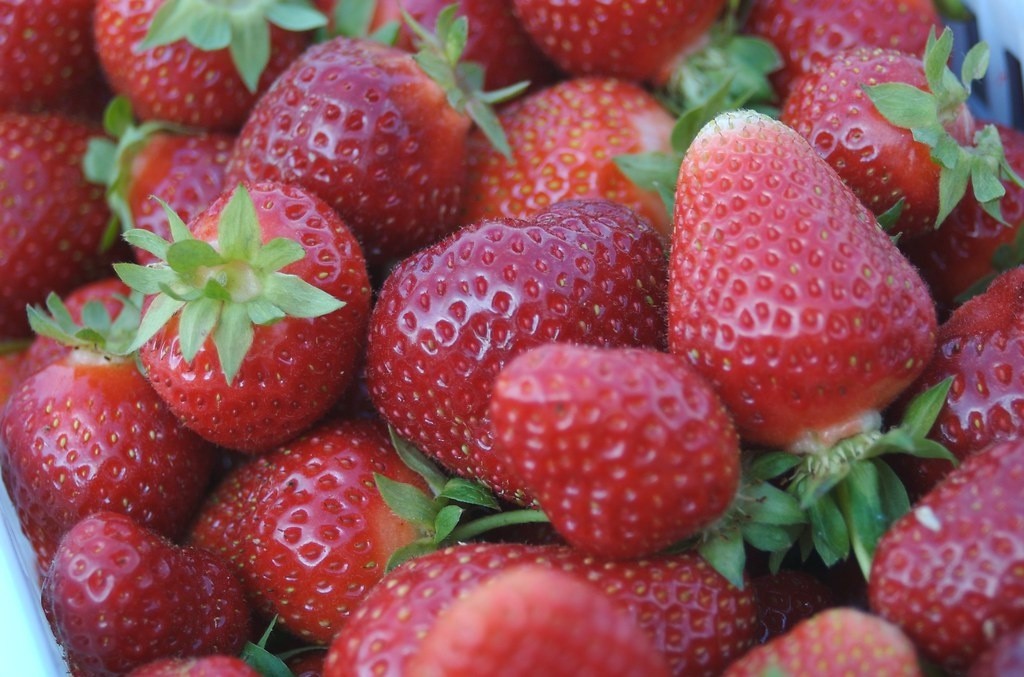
xmin=0 ymin=0 xmax=1024 ymax=677
